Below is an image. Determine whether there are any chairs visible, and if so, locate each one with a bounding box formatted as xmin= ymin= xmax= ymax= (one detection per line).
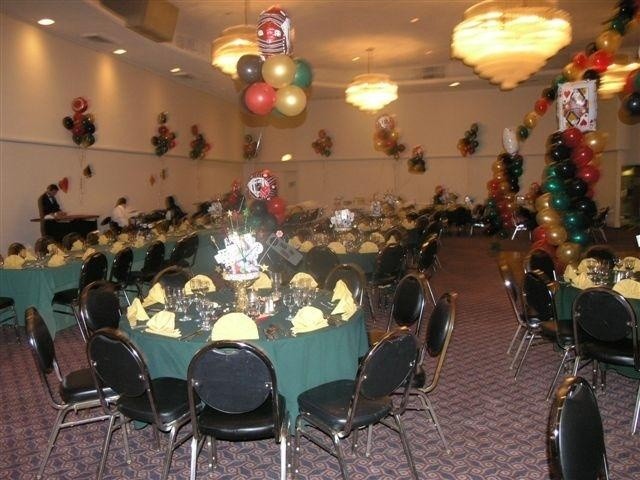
xmin=409 ymin=237 xmax=438 ymax=307
xmin=7 ymin=243 xmax=28 ymax=258
xmin=33 ymin=237 xmax=57 ymax=257
xmin=592 ymin=206 xmax=610 ymax=243
xmin=186 ymin=341 xmax=291 ymax=479
xmin=306 ymin=246 xmax=340 ymax=288
xmin=582 ymin=246 xmax=619 ymax=270
xmin=88 ymin=232 xmax=102 ymax=245
xmin=165 ymin=232 xmax=198 ymax=277
xmin=417 ymin=223 xmax=447 ymax=270
xmin=293 ymin=328 xmax=417 ymax=479
xmin=358 ymin=293 xmax=455 ymax=457
xmin=513 ymin=272 xmax=607 ymax=400
xmin=498 ymin=253 xmax=554 ymax=371
xmin=366 ymin=273 xmax=425 ymax=336
xmin=77 ymin=281 xmax=120 ymax=334
xmin=51 ymin=252 xmax=108 ymax=343
xmin=22 ymin=307 xmax=131 ymax=480
xmin=366 ymin=244 xmax=408 ymax=324
xmin=154 ymin=266 xmax=192 ymax=292
xmin=572 ymin=288 xmax=640 ymax=435
xmin=117 ymin=242 xmax=165 ymax=298
xmin=0 ymin=297 xmax=20 ymax=348
xmin=323 ymin=263 xmax=364 ymax=307
xmin=546 ymin=375 xmax=611 ymax=479
xmin=87 ymin=328 xmax=218 ymax=480
xmin=106 ymin=249 xmax=135 ymax=318
xmin=521 ymin=248 xmax=556 ymax=287
xmin=63 ymin=232 xmax=85 ymax=249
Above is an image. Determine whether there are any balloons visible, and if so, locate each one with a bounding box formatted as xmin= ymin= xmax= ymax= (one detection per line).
xmin=245 ymin=82 xmax=276 ymax=114
xmin=488 ymin=153 xmax=524 ymax=238
xmin=257 ymin=6 xmax=296 ymax=57
xmin=242 ymin=135 xmax=261 ymax=160
xmin=237 ymin=55 xmax=263 ymax=83
xmin=262 ymin=54 xmax=295 ymax=88
xmin=586 ymin=43 xmax=597 ymax=56
xmin=65 ymin=99 xmax=95 ymax=147
xmin=544 ymin=225 xmax=567 ymax=244
xmin=597 ymin=31 xmax=621 ymax=52
xmin=619 ymin=69 xmax=640 ymax=125
xmin=573 ymin=54 xmax=587 ymax=68
xmin=554 ymin=76 xmax=566 ymax=90
xmin=563 ymin=129 xmax=582 ymax=147
xmin=312 ymin=130 xmax=332 ymax=157
xmin=151 ymin=111 xmax=177 ymax=156
xmin=590 ymin=49 xmax=607 ymax=71
xmin=570 ymin=231 xmax=594 ymax=247
xmin=458 ymin=124 xmax=478 ymax=156
xmin=536 ymin=211 xmax=560 ymax=224
xmin=562 ymin=212 xmax=582 ymax=228
xmin=563 ymin=63 xmax=578 ymax=81
xmin=573 ymin=147 xmax=593 ymax=164
xmin=191 ymin=135 xmax=210 ymax=161
xmin=556 ymin=161 xmax=577 ymax=178
xmin=572 ymin=198 xmax=597 ymax=216
xmin=548 ymin=193 xmax=568 ymax=209
xmin=566 ymin=178 xmax=588 ymax=194
xmin=373 ymin=115 xmax=405 ymax=159
xmin=516 ymin=125 xmax=528 ymax=141
xmin=502 ymin=128 xmax=519 ymax=154
xmin=543 ymin=163 xmax=558 ymax=178
xmin=550 ymin=143 xmax=572 ymax=159
xmin=531 ymin=227 xmax=547 ymax=240
xmin=535 ymin=99 xmax=548 ymax=117
xmin=543 ymin=88 xmax=555 ymax=104
xmin=585 ymin=133 xmax=604 ymax=153
xmin=536 ymin=193 xmax=553 ymax=209
xmin=542 ymin=178 xmax=564 ymax=190
xmin=293 ymin=60 xmax=312 ymax=89
xmin=579 ymin=164 xmax=600 ymax=182
xmin=556 ymin=244 xmax=576 ymax=260
xmin=408 ymin=144 xmax=426 ymax=174
xmin=277 ymin=85 xmax=306 ymax=117
xmin=583 ymin=69 xmax=601 ymax=90
xmin=523 ymin=112 xmax=537 ymax=129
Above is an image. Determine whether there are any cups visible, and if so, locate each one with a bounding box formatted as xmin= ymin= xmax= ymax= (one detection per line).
xmin=190 ymin=279 xmax=200 ymax=298
xmin=179 ymin=298 xmax=192 ymax=321
xmin=200 ymin=281 xmax=210 ymax=297
xmin=283 ymin=294 xmax=298 ymax=321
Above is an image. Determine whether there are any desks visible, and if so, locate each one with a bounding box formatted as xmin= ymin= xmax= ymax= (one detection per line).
xmin=28 ymin=214 xmax=98 ymax=242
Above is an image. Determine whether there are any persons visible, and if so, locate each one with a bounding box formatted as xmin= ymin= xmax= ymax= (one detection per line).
xmin=112 ymin=198 xmax=128 ymax=226
xmin=165 ymin=196 xmax=182 ymax=220
xmin=38 ymin=184 xmax=61 ymax=238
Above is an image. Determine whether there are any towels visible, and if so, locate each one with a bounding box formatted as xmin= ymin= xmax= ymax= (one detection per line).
xmin=5 ymin=254 xmax=24 ymax=265
xmin=290 ymin=306 xmax=329 ymax=335
xmin=109 ymin=241 xmax=124 ymax=255
xmin=380 ymin=224 xmax=393 ymax=232
xmin=359 ymin=242 xmax=379 ymax=254
xmin=246 ymin=270 xmax=273 ymax=290
xmin=210 ymin=312 xmax=259 ymax=342
xmin=564 ymin=264 xmax=577 ymax=284
xmin=577 ymin=256 xmax=598 ymax=274
xmin=613 ymin=257 xmax=640 ymax=273
xmin=142 ymin=282 xmax=169 ymax=307
xmin=155 ymin=235 xmax=167 ymax=244
xmin=330 ymin=292 xmax=357 ymax=322
xmin=371 ymin=232 xmax=385 ymax=243
xmin=405 ymin=223 xmax=416 ymax=229
xmin=135 ymin=239 xmax=145 ymax=249
xmin=357 ymin=225 xmax=371 ymax=231
xmin=298 ymin=241 xmax=315 ymax=252
xmin=24 ymin=253 xmax=38 ymax=261
xmin=149 ymin=228 xmax=158 ymax=237
xmin=331 ymin=279 xmax=353 ymax=303
xmin=384 ymin=235 xmax=398 ymax=248
xmin=3 ymin=260 xmax=23 ymax=270
xmin=71 ymin=240 xmax=83 ymax=252
xmin=290 ymin=236 xmax=301 ymax=250
xmin=183 ymin=274 xmax=217 ymax=296
xmin=54 ymin=247 xmax=64 ymax=256
xmin=289 ymin=272 xmax=319 ymax=290
xmin=611 ymin=278 xmax=640 ymax=300
xmin=127 ymin=297 xmax=150 ymax=322
xmin=97 ymin=236 xmax=109 ymax=246
xmin=571 ymin=272 xmax=601 ymax=290
xmin=328 ymin=241 xmax=346 ymax=256
xmin=80 ymin=247 xmax=95 ymax=261
xmin=116 ymin=233 xmax=129 ymax=243
xmin=47 ymin=255 xmax=65 ymax=268
xmin=144 ymin=310 xmax=183 ymax=339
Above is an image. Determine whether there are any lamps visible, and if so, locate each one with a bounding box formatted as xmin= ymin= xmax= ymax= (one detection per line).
xmin=211 ymin=0 xmax=262 ymax=77
xmin=451 ymin=0 xmax=571 ymax=90
xmin=346 ymin=43 xmax=398 ymax=113
